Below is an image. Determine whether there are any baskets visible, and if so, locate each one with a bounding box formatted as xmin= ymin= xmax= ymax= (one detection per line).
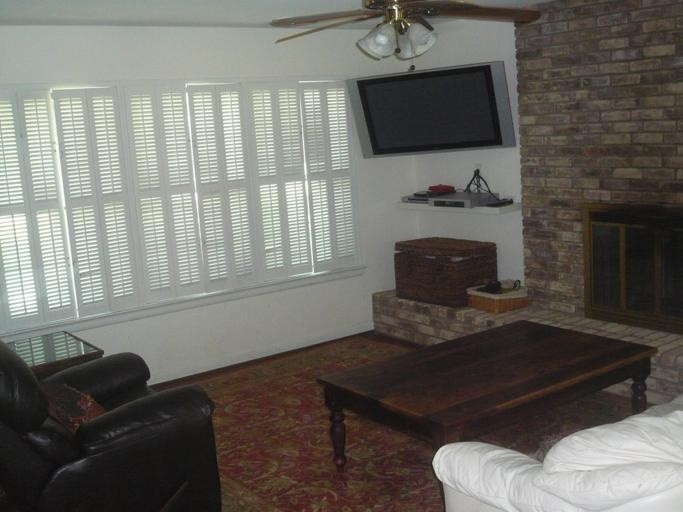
xmin=466 ymin=281 xmax=528 ymax=313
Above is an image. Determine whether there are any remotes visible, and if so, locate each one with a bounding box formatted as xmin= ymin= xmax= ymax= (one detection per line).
xmin=487 ymin=198 xmax=513 ymax=207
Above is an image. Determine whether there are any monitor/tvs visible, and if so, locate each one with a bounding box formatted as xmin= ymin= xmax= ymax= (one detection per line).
xmin=345 ymin=61 xmax=517 ymax=160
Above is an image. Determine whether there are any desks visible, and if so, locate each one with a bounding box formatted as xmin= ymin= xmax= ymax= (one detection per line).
xmin=7 ymin=330 xmax=104 ymax=379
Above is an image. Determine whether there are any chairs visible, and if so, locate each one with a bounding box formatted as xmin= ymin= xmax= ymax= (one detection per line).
xmin=2 ymin=342 xmax=223 ymax=511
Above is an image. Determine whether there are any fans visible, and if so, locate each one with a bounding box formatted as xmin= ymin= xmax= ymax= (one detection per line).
xmin=269 ymin=0 xmax=540 ymax=44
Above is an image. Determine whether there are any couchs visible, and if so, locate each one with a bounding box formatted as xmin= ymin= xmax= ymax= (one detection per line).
xmin=433 ymin=403 xmax=683 ymax=511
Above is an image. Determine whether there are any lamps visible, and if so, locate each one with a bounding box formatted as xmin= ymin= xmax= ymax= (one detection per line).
xmin=355 ymin=8 xmax=438 ymax=59
